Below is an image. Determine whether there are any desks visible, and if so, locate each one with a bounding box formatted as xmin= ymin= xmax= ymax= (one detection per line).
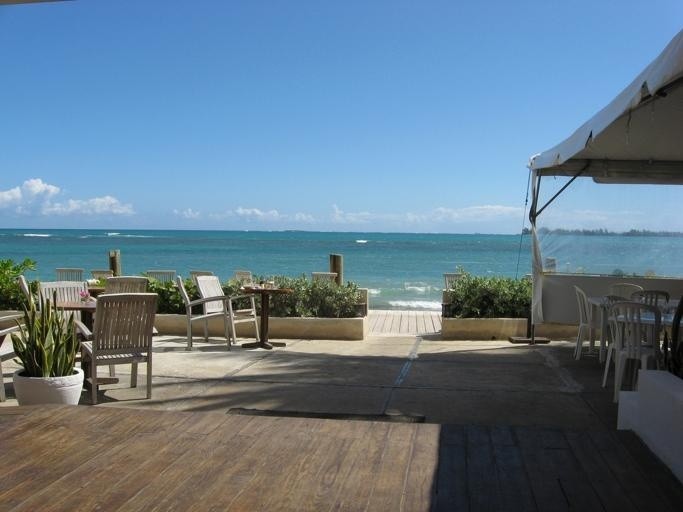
xmin=238 ymin=283 xmax=298 ymax=350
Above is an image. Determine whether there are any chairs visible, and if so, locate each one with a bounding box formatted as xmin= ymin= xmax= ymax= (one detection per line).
xmin=566 ymin=275 xmax=682 ymax=406
xmin=310 ymin=269 xmax=366 ymax=319
xmin=0 ymin=266 xmax=260 ymax=408
xmin=442 ymin=271 xmax=471 ymax=319
